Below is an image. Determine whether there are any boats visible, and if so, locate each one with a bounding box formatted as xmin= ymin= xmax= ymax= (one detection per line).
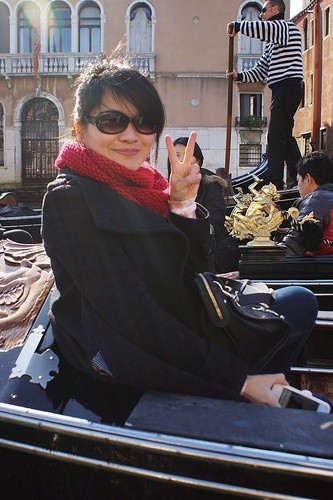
xmin=0 ymin=153 xmax=333 ymax=500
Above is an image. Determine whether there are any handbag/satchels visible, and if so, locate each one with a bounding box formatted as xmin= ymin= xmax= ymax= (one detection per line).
xmin=193 ymin=272 xmax=290 ymax=373
xmin=215 ymin=236 xmax=240 ymax=274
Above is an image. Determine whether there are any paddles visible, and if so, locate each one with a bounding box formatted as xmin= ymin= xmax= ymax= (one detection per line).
xmin=224 ymin=22 xmax=235 ymax=176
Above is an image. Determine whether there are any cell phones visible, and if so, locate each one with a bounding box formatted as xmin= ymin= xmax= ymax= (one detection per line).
xmin=271 ymin=383 xmax=331 ymax=414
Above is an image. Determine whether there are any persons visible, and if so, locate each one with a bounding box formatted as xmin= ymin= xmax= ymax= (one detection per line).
xmin=41 ymin=45 xmax=330 ymax=410
xmin=166 ymin=137 xmax=333 ymax=256
xmin=225 ymin=0 xmax=304 ymax=191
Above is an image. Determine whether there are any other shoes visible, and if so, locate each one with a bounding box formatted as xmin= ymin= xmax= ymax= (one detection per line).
xmin=286 ymin=181 xmax=297 ymax=189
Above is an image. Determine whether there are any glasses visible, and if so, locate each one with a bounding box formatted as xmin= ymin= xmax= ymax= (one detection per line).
xmin=86 ymin=111 xmax=158 ymax=135
xmin=260 ymin=4 xmax=279 ymax=13
